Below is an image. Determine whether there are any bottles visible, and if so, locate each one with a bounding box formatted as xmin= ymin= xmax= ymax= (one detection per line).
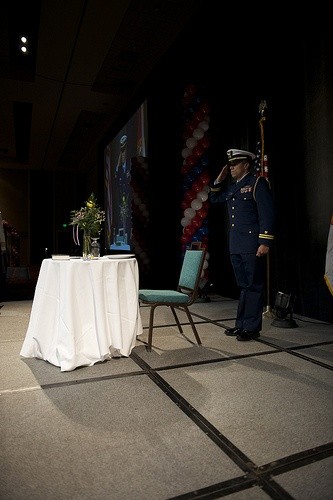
xmin=90 ymin=237 xmax=100 ymax=260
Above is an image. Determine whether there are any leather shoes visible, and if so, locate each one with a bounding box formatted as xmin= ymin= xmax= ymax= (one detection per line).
xmin=224 ymin=327 xmax=243 ymax=336
xmin=236 ymin=330 xmax=260 ymax=341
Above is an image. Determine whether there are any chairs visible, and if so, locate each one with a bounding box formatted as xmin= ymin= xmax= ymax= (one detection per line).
xmin=139 ymin=241 xmax=207 ymax=353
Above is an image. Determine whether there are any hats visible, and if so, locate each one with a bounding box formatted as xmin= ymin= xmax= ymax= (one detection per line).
xmin=226 ymin=148 xmax=256 ymax=166
xmin=119 ymin=135 xmax=128 ymax=150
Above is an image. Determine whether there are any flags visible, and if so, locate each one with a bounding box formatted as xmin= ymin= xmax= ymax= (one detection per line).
xmin=106 ymin=144 xmax=112 ymax=246
xmin=254 ymin=143 xmax=268 ymax=182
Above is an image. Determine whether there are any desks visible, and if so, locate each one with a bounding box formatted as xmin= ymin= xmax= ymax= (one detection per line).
xmin=19 ymin=257 xmax=143 ymax=371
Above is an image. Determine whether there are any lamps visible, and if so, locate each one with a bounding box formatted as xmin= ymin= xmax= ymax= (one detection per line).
xmin=198 ymin=279 xmax=213 ymax=300
xmin=272 ymin=290 xmax=298 ymax=327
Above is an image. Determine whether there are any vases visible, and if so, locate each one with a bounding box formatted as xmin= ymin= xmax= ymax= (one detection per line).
xmin=82 ymin=230 xmax=93 ymax=259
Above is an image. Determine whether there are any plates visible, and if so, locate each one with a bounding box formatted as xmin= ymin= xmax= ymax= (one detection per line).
xmin=104 ymin=254 xmax=135 ymax=258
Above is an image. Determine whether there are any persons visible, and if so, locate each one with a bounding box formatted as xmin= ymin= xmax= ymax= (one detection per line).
xmin=210 ymin=149 xmax=275 ymax=341
xmin=114 ymin=135 xmax=131 ymax=232
xmin=0 ymin=209 xmax=18 ymax=294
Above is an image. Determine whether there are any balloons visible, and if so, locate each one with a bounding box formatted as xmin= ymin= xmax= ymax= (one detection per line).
xmin=181 ymin=84 xmax=210 ymax=280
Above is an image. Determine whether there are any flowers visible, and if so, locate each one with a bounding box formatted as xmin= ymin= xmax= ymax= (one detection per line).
xmin=68 ymin=195 xmax=103 ymax=253
